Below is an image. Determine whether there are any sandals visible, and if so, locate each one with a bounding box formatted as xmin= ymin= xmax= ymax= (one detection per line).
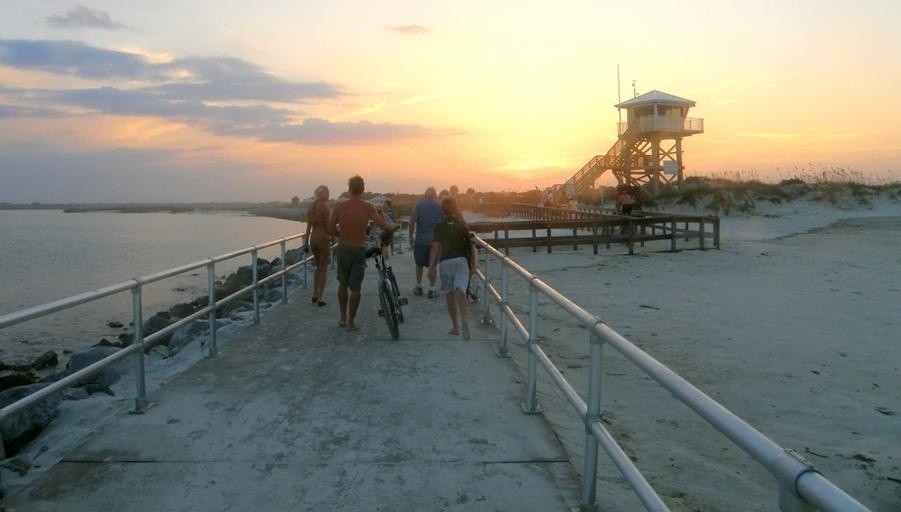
xmin=312 ymin=296 xmax=327 ymax=307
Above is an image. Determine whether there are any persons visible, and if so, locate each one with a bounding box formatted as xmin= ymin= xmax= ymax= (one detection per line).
xmin=409 ymin=187 xmax=442 ymax=298
xmin=376 ymin=209 xmax=394 ymax=260
xmin=616 ymin=189 xmax=633 ymax=216
xmin=384 ymin=199 xmax=398 ymax=256
xmin=426 ymin=196 xmax=474 ymax=341
xmin=536 ymin=189 xmax=579 ymax=211
xmin=328 ymin=174 xmax=400 ymax=327
xmin=303 ymin=184 xmax=331 ymax=307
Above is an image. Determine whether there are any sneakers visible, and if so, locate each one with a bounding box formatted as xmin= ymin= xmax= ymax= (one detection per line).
xmin=413 ymin=287 xmax=439 ymax=298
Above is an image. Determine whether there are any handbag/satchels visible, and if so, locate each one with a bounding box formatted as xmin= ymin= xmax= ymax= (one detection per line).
xmin=469 ymin=245 xmax=478 ymax=270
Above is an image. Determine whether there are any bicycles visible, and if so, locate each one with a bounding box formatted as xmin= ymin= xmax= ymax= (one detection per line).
xmin=364 ymin=225 xmax=408 ymax=341
